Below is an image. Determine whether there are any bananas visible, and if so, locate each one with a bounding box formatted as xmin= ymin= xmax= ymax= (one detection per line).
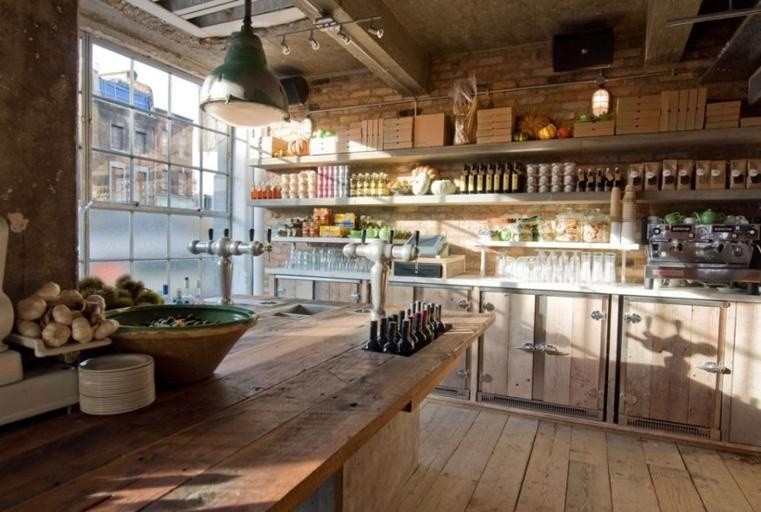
xmin=273 ymin=149 xmax=289 ymax=157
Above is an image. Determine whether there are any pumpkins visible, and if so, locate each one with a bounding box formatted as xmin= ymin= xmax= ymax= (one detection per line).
xmin=410 ymin=165 xmax=440 ymax=180
xmin=518 ymin=113 xmax=549 ymax=139
xmin=411 ymin=171 xmax=430 ymax=195
xmin=514 ymin=133 xmax=529 ymax=142
xmin=537 ymin=124 xmax=557 ymax=138
xmin=431 ymin=179 xmax=457 ymax=195
xmin=287 ymin=138 xmax=308 ymax=155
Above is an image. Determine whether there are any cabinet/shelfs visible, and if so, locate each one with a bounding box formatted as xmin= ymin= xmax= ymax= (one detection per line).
xmin=247 ymin=125 xmax=761 ymax=456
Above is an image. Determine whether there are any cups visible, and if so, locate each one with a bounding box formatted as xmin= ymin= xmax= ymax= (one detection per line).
xmin=494 ymin=184 xmax=637 ymax=287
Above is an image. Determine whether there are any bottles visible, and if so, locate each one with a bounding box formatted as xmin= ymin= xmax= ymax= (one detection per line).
xmin=249 ymin=164 xmax=390 ymax=200
xmin=459 ymin=159 xmax=526 ymax=195
xmin=363 ymin=300 xmax=446 ymax=357
xmin=576 ymin=166 xmax=622 ymax=192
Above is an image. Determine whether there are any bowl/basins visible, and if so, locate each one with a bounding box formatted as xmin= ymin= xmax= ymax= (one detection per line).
xmin=101 ymin=303 xmax=260 ymax=385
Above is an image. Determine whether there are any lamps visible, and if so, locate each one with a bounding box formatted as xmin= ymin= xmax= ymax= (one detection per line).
xmin=201 ymin=0 xmax=289 ymax=128
xmin=202 ymin=16 xmax=385 ymax=55
xmin=591 ymin=85 xmax=611 ymax=118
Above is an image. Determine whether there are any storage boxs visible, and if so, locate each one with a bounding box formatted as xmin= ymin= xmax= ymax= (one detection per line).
xmin=414 ymin=102 xmax=446 ymax=149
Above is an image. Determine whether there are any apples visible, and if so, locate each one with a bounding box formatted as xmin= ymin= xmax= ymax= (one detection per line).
xmin=557 ymin=127 xmax=569 ymax=138
xmin=314 ymin=129 xmax=334 ymax=138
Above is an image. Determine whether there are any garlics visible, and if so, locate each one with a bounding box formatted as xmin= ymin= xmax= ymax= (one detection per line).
xmin=13 ymin=280 xmax=119 ymax=348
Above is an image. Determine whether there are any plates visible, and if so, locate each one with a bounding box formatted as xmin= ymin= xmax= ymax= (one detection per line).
xmin=77 ymin=351 xmax=157 ymax=416
